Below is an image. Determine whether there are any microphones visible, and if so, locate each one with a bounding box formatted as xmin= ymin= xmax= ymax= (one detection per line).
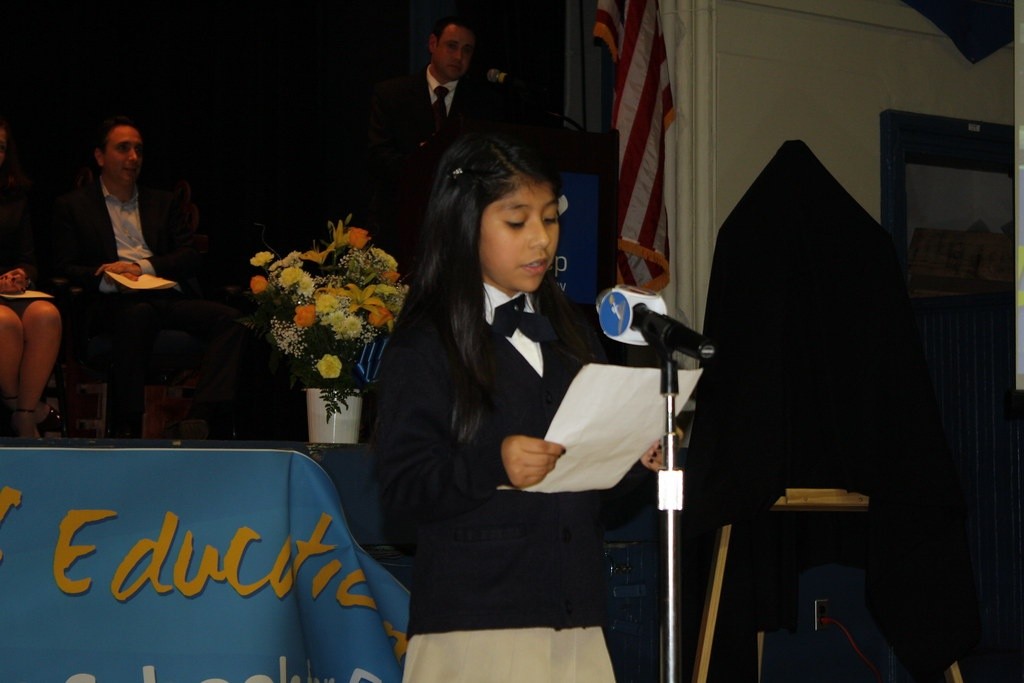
xmin=594 ymin=283 xmax=716 ymax=362
xmin=487 ymin=69 xmax=516 ymax=86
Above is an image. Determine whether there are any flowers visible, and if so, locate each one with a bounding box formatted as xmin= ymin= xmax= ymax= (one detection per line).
xmin=241 ymin=212 xmax=409 ymax=424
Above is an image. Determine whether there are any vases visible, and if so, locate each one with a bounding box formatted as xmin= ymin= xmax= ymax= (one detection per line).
xmin=303 ymin=388 xmax=362 ymax=445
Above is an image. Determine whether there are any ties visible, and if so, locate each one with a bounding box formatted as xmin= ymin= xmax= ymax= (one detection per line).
xmin=431 ymin=86 xmax=449 ymax=134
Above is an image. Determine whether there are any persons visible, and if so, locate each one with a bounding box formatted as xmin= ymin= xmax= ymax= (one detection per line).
xmin=53 ymin=117 xmax=250 ymax=441
xmin=0 ymin=121 xmax=62 ymax=442
xmin=378 ymin=16 xmax=489 ymax=257
xmin=369 ymin=124 xmax=684 ymax=651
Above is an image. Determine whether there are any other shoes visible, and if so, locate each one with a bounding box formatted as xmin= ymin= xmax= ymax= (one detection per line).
xmin=160 ymin=420 xmax=209 ymax=440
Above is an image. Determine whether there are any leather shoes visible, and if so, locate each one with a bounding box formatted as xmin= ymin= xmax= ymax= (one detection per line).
xmin=5 ymin=397 xmax=63 ymax=433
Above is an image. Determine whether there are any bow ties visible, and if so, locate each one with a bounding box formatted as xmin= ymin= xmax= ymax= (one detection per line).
xmin=489 ymin=295 xmax=559 ymax=344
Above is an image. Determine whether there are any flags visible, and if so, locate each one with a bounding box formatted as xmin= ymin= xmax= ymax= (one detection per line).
xmin=591 ymin=0 xmax=677 ymax=291
